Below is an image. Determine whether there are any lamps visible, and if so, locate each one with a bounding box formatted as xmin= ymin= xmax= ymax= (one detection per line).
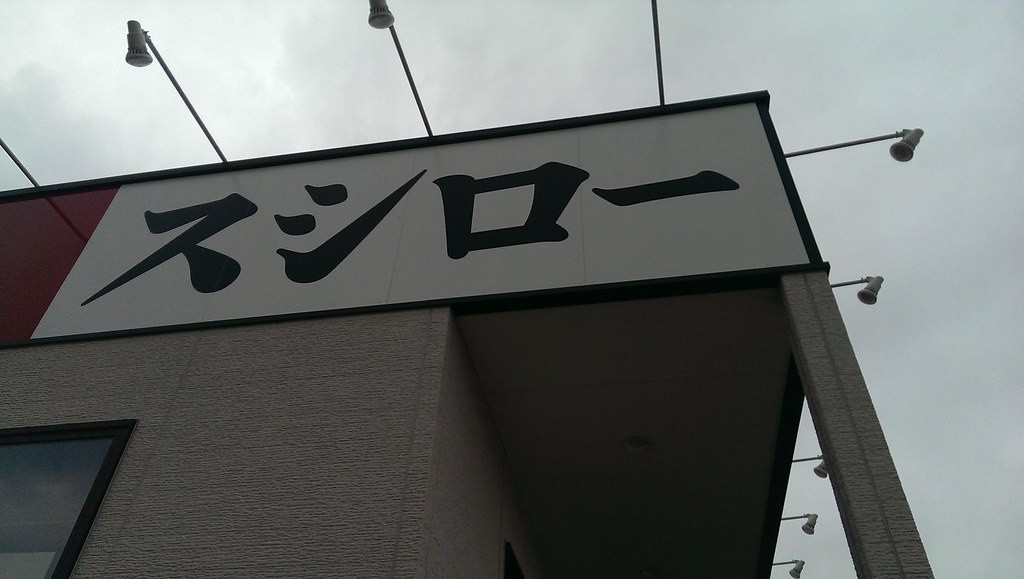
xmin=369 ymin=0 xmax=433 ymax=137
xmin=781 ymin=514 xmax=818 ymax=534
xmin=831 ymin=276 xmax=884 ymax=305
xmin=773 ymin=559 xmax=806 ymax=579
xmin=792 ymin=455 xmax=827 ymax=478
xmin=126 ymin=19 xmax=228 ymax=163
xmin=785 ymin=128 xmax=924 ymax=162
